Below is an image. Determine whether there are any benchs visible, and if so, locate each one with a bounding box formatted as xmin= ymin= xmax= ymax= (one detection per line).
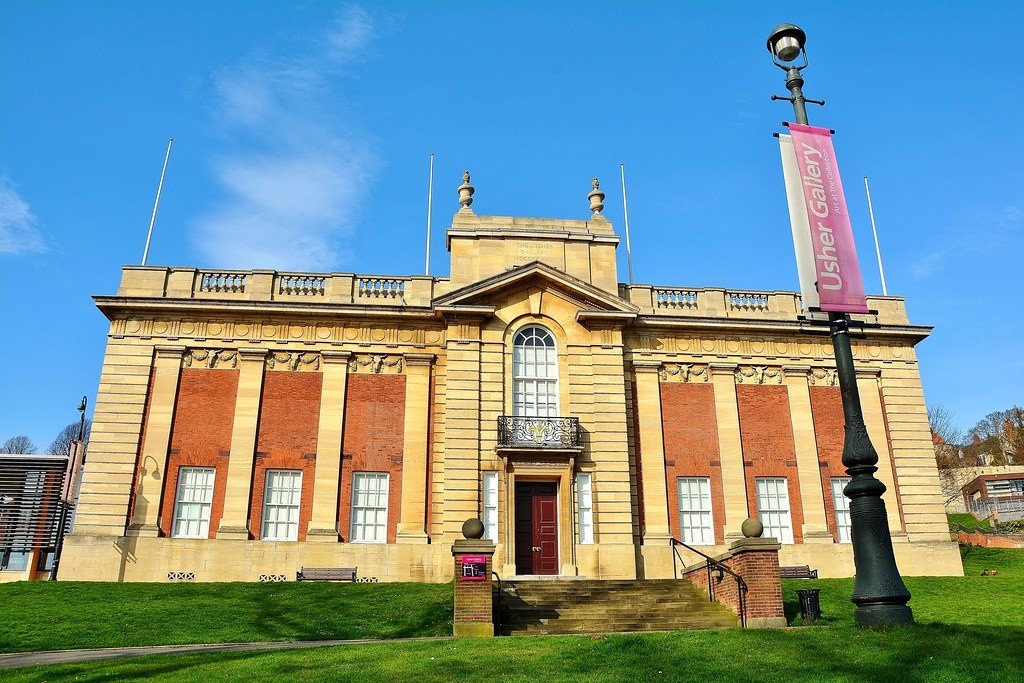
xmin=779 ymin=565 xmax=817 ymax=578
xmin=295 ymin=566 xmax=357 ymax=582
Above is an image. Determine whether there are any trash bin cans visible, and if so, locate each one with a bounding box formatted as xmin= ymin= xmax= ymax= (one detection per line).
xmin=795 ymin=588 xmax=823 ymax=621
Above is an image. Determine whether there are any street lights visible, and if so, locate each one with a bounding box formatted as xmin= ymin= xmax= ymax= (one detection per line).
xmin=47 ymin=396 xmax=89 ymax=582
xmin=766 ymin=22 xmax=916 ymax=632
